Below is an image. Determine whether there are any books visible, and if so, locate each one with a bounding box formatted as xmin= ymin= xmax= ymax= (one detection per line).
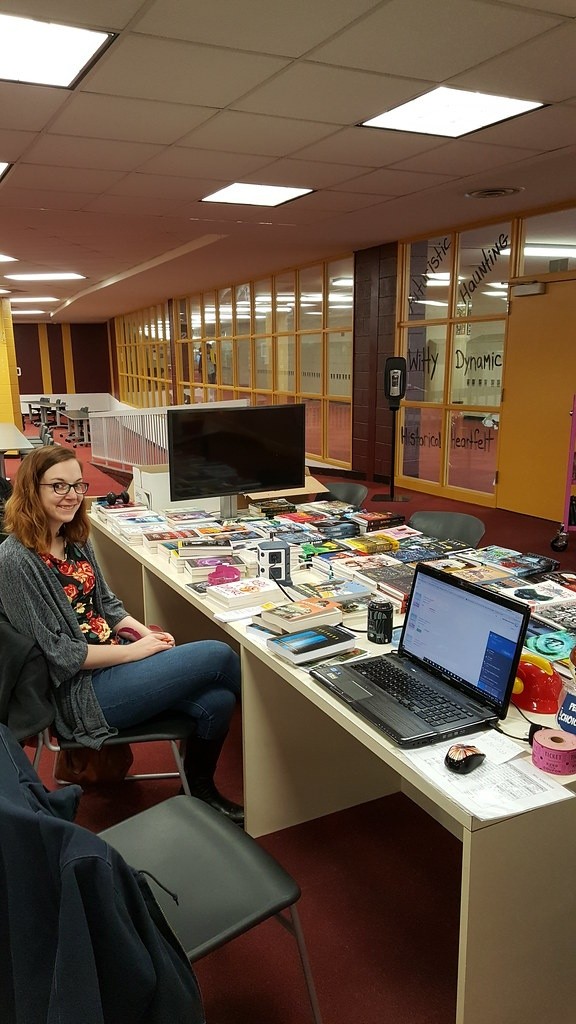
xmin=222 ymin=496 xmax=415 ymax=620
xmin=89 ymin=497 xmax=174 ymax=547
xmin=142 ymin=528 xmax=372 ymax=674
xmin=303 ymin=499 xmax=576 ymax=659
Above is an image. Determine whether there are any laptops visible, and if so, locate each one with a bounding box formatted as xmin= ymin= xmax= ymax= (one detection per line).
xmin=308 ymin=562 xmax=531 ymax=748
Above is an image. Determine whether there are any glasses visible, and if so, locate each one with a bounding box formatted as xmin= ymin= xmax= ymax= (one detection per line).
xmin=38 ymin=482 xmax=90 ymax=496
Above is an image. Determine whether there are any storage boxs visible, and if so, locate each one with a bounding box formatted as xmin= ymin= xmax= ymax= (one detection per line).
xmin=237 ymin=464 xmax=330 ymax=508
xmin=131 ymin=463 xmax=220 ymax=509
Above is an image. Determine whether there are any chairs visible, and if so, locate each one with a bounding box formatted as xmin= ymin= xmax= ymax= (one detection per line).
xmin=20 ymin=423 xmax=54 ymax=461
xmin=315 ymin=483 xmax=368 ymax=508
xmin=0 ymin=614 xmax=192 ymax=795
xmin=32 ymin=398 xmax=91 ymax=442
xmin=0 ymin=724 xmax=325 ymax=1024
xmin=405 ymin=511 xmax=485 ymax=549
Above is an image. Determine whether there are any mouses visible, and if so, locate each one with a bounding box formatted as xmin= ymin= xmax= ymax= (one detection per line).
xmin=444 ymin=743 xmax=486 ymax=774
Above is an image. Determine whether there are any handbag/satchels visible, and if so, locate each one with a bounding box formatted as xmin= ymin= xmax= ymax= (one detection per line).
xmin=53 ymin=744 xmax=133 ymax=787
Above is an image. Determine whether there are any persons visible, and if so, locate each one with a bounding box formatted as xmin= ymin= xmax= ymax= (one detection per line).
xmin=198 ymin=344 xmax=217 ymax=384
xmin=1 ymin=445 xmax=244 ymax=826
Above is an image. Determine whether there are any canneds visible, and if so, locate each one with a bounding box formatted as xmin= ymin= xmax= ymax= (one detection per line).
xmin=367 ymin=598 xmax=393 ymax=645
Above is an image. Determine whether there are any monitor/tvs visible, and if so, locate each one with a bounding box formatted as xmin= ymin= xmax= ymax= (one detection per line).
xmin=166 ymin=403 xmax=306 ymax=521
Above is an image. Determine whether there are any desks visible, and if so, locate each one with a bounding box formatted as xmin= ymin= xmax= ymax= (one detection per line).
xmin=87 ymin=504 xmax=575 ymax=1024
xmin=0 ymin=421 xmax=36 ymax=481
xmin=21 ymin=401 xmax=68 ymax=430
xmin=57 ymin=409 xmax=92 ymax=447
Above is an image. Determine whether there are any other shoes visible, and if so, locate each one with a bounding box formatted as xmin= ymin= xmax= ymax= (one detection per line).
xmin=178 ymin=780 xmax=245 ymax=826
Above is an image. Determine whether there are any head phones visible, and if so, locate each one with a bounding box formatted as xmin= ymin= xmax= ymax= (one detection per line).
xmin=97 ymin=491 xmax=129 ymax=505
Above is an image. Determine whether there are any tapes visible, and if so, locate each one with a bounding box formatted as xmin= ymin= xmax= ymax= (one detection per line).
xmin=532 ymin=728 xmax=576 ymax=775
